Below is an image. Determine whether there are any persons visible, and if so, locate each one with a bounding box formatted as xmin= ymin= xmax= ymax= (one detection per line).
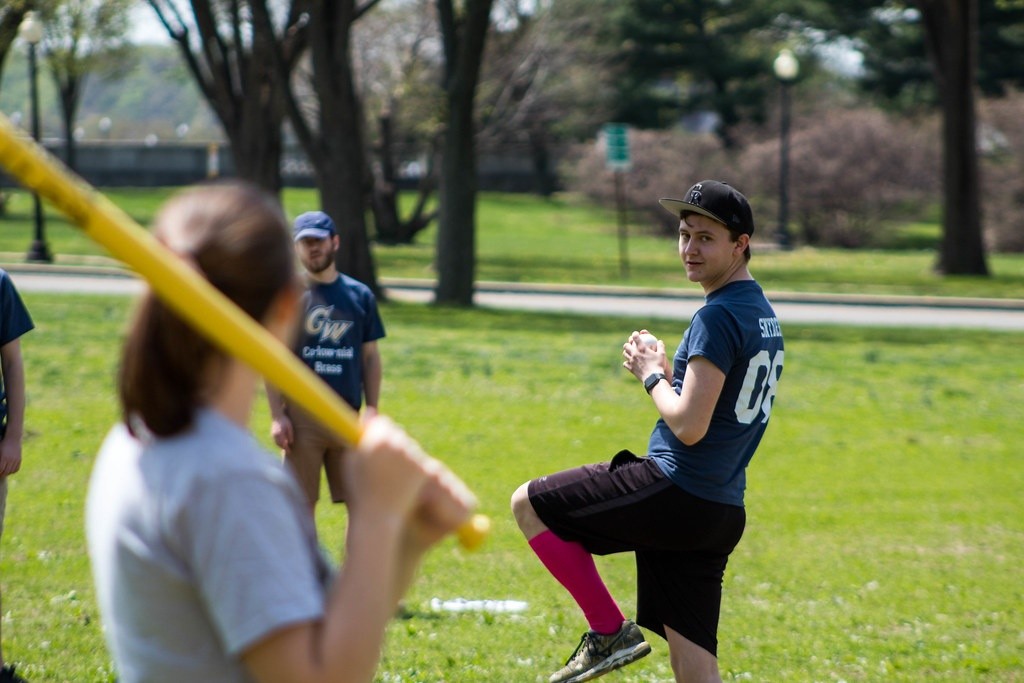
xmin=262 ymin=209 xmax=388 ymax=523
xmin=512 ymin=180 xmax=785 ymax=683
xmin=83 ymin=179 xmax=478 ymax=683
xmin=0 ymin=266 xmax=36 ymax=683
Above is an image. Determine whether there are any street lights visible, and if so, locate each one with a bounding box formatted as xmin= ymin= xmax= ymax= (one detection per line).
xmin=18 ymin=11 xmax=58 ymax=265
xmin=772 ymin=44 xmax=802 ymax=250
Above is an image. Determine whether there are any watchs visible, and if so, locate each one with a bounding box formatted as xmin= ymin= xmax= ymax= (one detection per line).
xmin=643 ymin=373 xmax=667 ymax=395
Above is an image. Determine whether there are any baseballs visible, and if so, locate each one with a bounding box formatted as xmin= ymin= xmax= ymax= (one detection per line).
xmin=633 ymin=334 xmax=659 ymax=352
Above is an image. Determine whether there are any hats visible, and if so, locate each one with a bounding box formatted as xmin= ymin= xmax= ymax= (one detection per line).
xmin=292 ymin=211 xmax=335 ymax=241
xmin=659 ymin=180 xmax=754 ymax=239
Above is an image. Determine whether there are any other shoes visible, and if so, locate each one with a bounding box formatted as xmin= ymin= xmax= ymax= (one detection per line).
xmin=0 ymin=663 xmax=28 ymax=683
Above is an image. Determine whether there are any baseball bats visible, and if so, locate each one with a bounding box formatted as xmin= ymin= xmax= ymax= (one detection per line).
xmin=0 ymin=115 xmax=492 ymax=551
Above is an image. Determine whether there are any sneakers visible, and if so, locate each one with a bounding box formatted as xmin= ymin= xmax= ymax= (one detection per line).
xmin=548 ymin=619 xmax=651 ymax=683
